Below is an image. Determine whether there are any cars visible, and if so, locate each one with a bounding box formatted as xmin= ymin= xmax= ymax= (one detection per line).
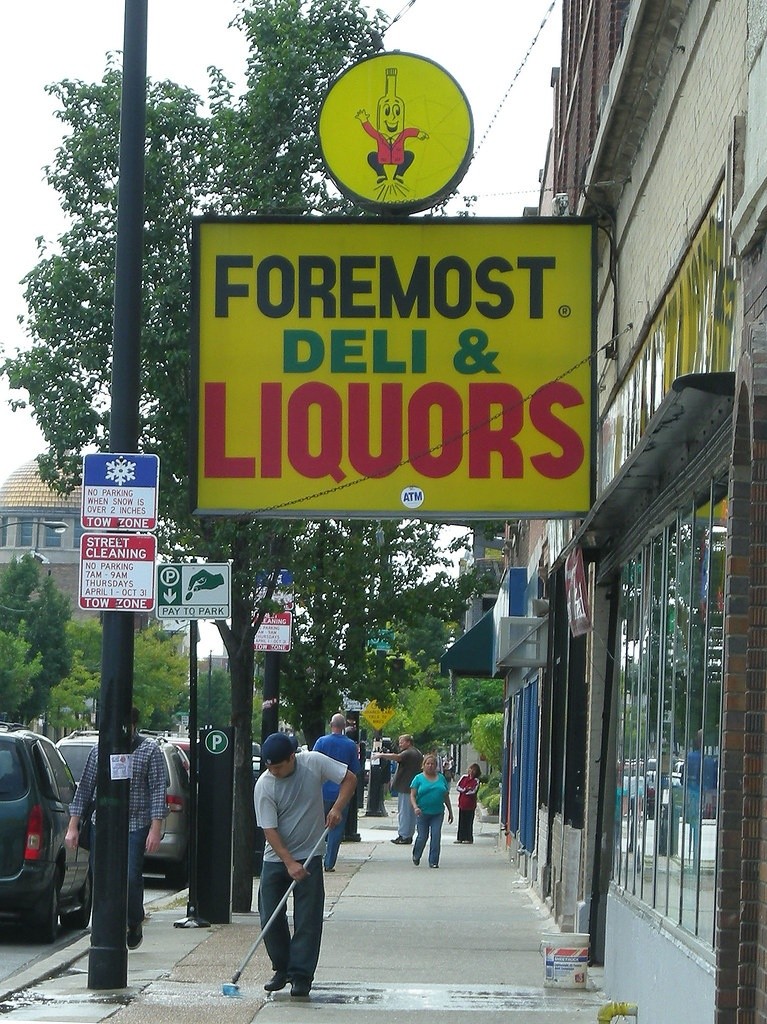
xmin=621 ymin=755 xmax=685 ymax=820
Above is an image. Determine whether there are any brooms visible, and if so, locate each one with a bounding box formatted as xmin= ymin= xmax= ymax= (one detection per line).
xmin=220 ymin=826 xmax=333 ymax=1000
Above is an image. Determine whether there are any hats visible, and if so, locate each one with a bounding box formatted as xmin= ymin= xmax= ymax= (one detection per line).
xmin=262 ymin=733 xmax=298 ymax=765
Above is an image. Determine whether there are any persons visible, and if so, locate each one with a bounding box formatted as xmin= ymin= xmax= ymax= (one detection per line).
xmin=254 ymin=733 xmax=359 ymax=997
xmin=410 ymin=754 xmax=454 ymax=868
xmin=442 ymin=756 xmax=457 ymax=781
xmin=312 ymin=714 xmax=358 ymax=872
xmin=616 ymin=729 xmax=717 ymax=852
xmin=65 ymin=706 xmax=166 ymax=950
xmin=453 ymin=763 xmax=482 ymax=844
xmin=371 ymin=734 xmax=424 ymax=844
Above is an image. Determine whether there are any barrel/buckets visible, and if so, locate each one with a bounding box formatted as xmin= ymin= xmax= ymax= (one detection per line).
xmin=539 ymin=933 xmax=590 ymax=990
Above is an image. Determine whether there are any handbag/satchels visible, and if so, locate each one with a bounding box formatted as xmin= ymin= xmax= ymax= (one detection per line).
xmin=78 ymin=797 xmax=95 ymax=851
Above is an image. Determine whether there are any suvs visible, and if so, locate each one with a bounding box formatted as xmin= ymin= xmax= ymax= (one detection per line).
xmin=55 ymin=729 xmax=192 ymax=889
xmin=0 ymin=718 xmax=95 ymax=948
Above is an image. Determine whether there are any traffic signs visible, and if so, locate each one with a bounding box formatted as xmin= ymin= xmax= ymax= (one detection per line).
xmin=152 ymin=561 xmax=234 ymax=622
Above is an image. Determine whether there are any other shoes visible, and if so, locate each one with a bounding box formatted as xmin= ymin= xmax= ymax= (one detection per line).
xmin=413 ymin=860 xmax=420 ymax=866
xmin=325 ymin=868 xmax=335 ymax=872
xmin=454 ymin=840 xmax=473 ymax=844
xmin=429 ymin=863 xmax=438 ymax=868
xmin=391 ymin=836 xmax=412 ymax=844
xmin=127 ymin=922 xmax=143 ymax=950
xmin=264 ymin=968 xmax=293 ymax=991
xmin=292 ymin=977 xmax=311 ymax=996
